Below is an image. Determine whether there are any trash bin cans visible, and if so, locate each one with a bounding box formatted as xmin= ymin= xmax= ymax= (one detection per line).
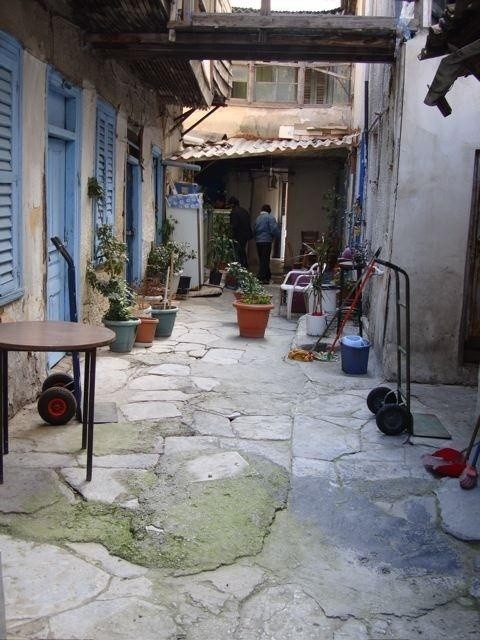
xmin=319 ymin=284 xmax=341 ymax=312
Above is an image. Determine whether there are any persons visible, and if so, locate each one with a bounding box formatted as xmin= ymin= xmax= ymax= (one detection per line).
xmin=228 ymin=197 xmax=253 ymax=269
xmin=252 ymin=204 xmax=281 ymax=285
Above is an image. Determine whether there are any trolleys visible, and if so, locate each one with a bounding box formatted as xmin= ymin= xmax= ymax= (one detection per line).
xmin=38 ymin=234 xmax=119 ymax=424
xmin=366 ymin=258 xmax=453 ymax=440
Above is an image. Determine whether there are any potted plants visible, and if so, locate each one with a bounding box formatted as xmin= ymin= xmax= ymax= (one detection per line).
xmin=224 ymin=261 xmax=274 ymax=337
xmin=304 ymin=185 xmax=347 ymax=337
xmin=83 ymin=177 xmax=141 ymax=352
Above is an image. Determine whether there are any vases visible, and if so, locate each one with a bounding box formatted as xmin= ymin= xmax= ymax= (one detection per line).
xmin=150 ymin=306 xmax=179 ymax=337
xmin=226 ymin=272 xmax=236 ymax=287
xmin=136 ymin=316 xmax=160 ymax=343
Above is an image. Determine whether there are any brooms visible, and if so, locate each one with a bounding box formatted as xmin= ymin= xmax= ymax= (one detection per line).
xmin=459 ymin=441 xmax=480 ymax=490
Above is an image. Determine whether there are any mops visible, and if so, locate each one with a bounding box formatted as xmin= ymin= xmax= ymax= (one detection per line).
xmin=288 ymin=245 xmax=383 ymax=361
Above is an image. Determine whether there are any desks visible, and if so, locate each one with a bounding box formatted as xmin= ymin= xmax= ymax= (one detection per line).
xmin=212 ymin=208 xmax=232 ymax=231
xmin=0 ymin=319 xmax=116 ymax=482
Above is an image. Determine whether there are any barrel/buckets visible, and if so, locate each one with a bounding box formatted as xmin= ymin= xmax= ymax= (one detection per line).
xmin=339 ymin=335 xmax=372 ymax=374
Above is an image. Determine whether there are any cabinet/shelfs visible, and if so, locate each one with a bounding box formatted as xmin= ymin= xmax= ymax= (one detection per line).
xmin=337 ymin=256 xmax=365 ymax=337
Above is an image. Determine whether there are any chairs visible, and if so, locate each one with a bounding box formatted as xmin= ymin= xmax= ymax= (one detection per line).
xmin=299 ymin=230 xmax=319 ymax=261
xmin=280 ymin=262 xmax=326 ymax=321
